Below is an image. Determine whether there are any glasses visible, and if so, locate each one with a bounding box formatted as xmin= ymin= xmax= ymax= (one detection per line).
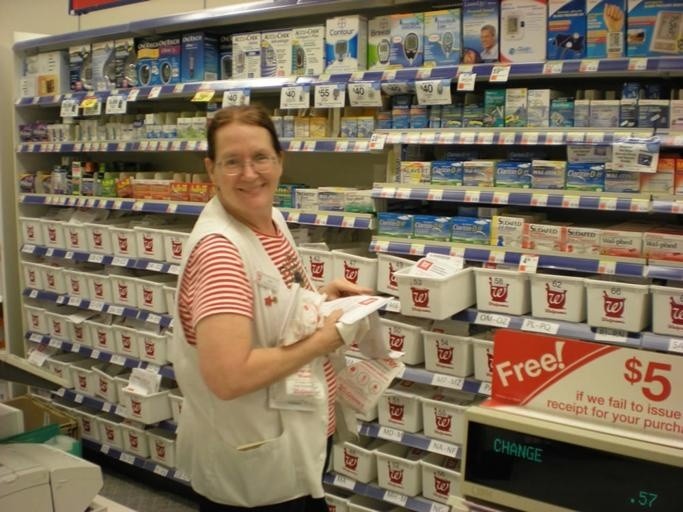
xmin=215 ymin=155 xmax=275 ymax=175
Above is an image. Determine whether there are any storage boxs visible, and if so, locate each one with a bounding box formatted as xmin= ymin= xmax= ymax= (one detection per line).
xmin=0 ymin=394 xmax=84 ymax=458
xmin=20 ymin=215 xmax=682 ymax=512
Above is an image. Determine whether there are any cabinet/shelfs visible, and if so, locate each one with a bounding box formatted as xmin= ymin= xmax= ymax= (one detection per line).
xmin=10 ymin=0 xmax=682 ymax=512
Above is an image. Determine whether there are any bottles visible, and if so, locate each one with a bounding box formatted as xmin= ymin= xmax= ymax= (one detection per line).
xmin=82 ymin=161 xmax=106 ymax=196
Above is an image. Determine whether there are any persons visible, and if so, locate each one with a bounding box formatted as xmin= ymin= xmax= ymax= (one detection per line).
xmin=626 ymin=27 xmax=646 ymax=46
xmin=602 ymin=3 xmax=629 ymax=59
xmin=475 ymin=25 xmax=498 ymax=61
xmin=167 ymin=101 xmax=376 ymax=512
